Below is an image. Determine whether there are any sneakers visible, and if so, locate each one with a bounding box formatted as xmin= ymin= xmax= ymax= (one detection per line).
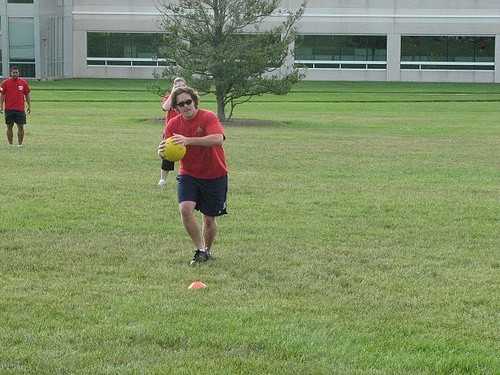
xmin=190 ymin=247 xmax=212 ymax=267
xmin=157 ymin=179 xmax=167 ymax=185
xmin=18 ymin=144 xmax=23 ymax=148
xmin=9 ymin=144 xmax=14 ymax=148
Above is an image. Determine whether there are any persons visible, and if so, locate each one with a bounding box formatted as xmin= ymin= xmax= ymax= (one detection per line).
xmin=0 ymin=67 xmax=31 ymax=148
xmin=156 ymin=77 xmax=185 ymax=186
xmin=157 ymin=86 xmax=230 ymax=268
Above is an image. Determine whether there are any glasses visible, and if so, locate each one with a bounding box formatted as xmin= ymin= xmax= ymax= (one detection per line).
xmin=176 ymin=99 xmax=193 ymax=107
xmin=13 ymin=72 xmax=18 ymax=74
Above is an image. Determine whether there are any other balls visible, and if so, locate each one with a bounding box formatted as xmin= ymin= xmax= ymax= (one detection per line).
xmin=162 ymin=137 xmax=186 ymax=162
